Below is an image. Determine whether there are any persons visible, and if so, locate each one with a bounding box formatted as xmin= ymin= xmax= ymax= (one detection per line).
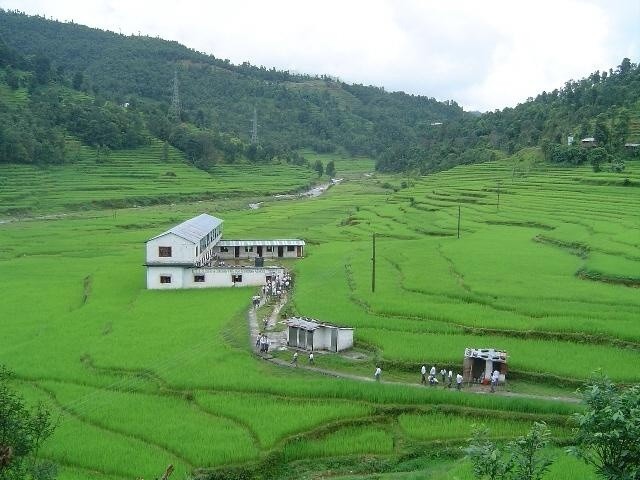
xmin=249 ymin=271 xmax=502 ymax=394
